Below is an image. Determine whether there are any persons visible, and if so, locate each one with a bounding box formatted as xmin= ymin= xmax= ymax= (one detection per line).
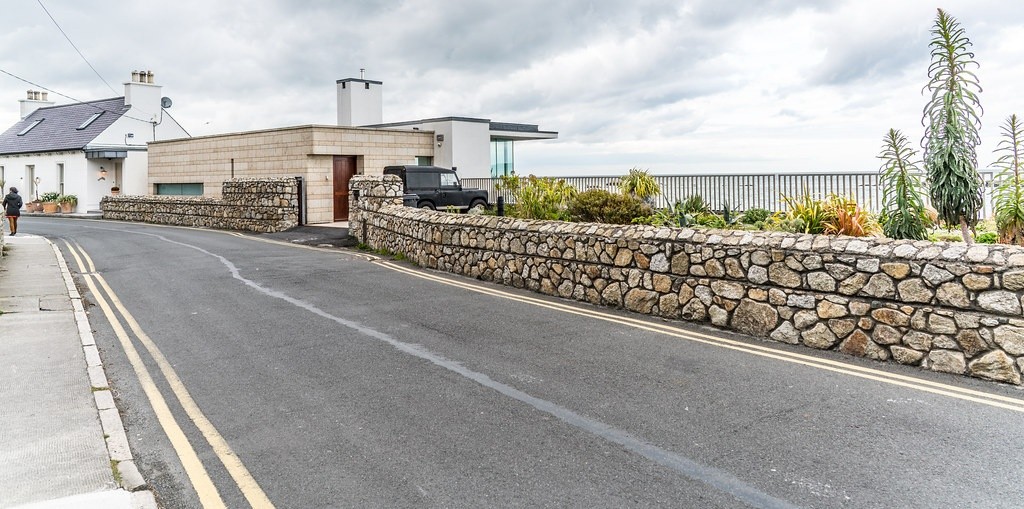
xmin=3 ymin=187 xmax=22 ymax=235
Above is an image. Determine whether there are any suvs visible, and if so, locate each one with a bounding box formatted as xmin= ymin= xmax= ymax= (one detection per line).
xmin=383 ymin=165 xmax=488 ymax=215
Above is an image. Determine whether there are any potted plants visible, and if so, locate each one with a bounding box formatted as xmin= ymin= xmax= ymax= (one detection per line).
xmin=57 ymin=194 xmax=78 ymax=214
xmin=40 ymin=192 xmax=60 ymax=213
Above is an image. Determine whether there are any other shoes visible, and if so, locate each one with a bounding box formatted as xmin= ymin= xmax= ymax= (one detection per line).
xmin=9 ymin=232 xmax=14 ymax=236
